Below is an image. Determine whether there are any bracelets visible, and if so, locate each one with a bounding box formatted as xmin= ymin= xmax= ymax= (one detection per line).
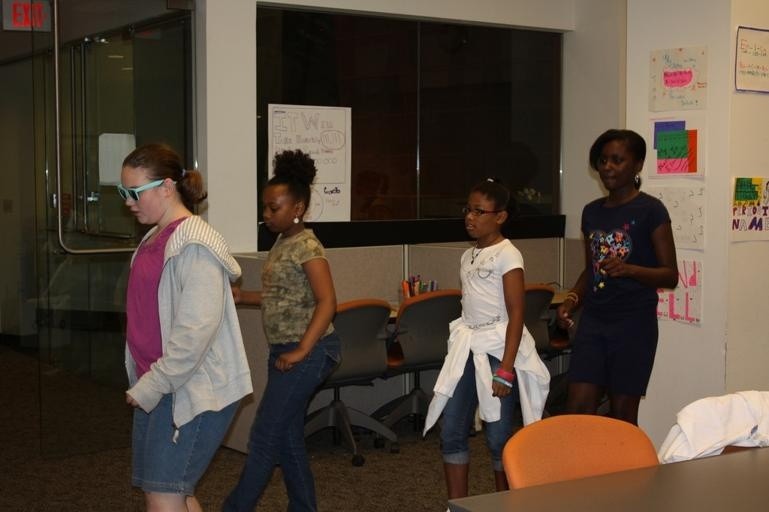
xmin=492 ymin=375 xmax=513 ymax=389
xmin=566 ymin=287 xmax=582 ymax=307
xmin=496 ymin=366 xmax=515 ymax=382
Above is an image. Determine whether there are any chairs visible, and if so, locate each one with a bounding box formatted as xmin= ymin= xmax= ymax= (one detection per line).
xmin=664 ymin=391 xmax=768 ymax=461
xmin=310 ymin=284 xmax=574 ymax=465
xmin=501 ymin=413 xmax=660 ymax=488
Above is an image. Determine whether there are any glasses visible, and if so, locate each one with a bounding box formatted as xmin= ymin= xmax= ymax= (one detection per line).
xmin=117 ymin=179 xmax=178 ymax=200
xmin=460 ymin=206 xmax=507 ymax=216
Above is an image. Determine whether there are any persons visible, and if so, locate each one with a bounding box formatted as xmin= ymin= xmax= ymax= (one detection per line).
xmin=421 ymin=171 xmax=552 ymax=512
xmin=115 ymin=141 xmax=255 ymax=512
xmin=555 ymin=127 xmax=679 ymax=429
xmin=220 ymin=147 xmax=343 ymax=512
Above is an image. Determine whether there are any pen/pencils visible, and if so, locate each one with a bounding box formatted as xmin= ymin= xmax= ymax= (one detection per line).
xmin=403 ymin=275 xmax=438 ymax=299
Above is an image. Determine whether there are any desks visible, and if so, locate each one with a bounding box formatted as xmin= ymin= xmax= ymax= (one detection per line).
xmin=444 ymin=441 xmax=769 ymax=512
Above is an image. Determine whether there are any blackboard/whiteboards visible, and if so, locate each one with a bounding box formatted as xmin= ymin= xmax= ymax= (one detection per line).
xmin=254 ymin=1 xmax=566 ymax=252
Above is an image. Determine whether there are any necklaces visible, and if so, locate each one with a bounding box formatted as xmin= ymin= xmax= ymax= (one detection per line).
xmin=471 ymin=233 xmax=501 ymax=265
